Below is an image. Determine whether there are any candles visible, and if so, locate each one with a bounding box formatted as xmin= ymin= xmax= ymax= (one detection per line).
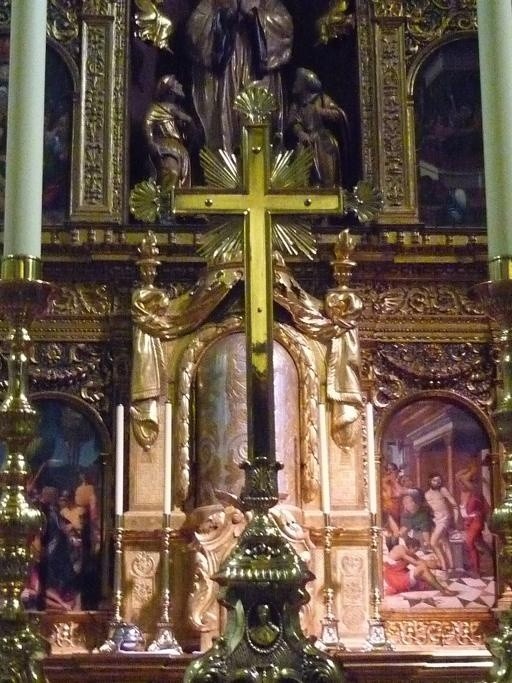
xmin=473 ymin=1 xmax=512 ymax=256
xmin=319 ymin=402 xmax=330 ymax=512
xmin=165 ymin=403 xmax=172 ymax=512
xmin=365 ymin=400 xmax=379 ymax=512
xmin=114 ymin=402 xmax=124 ymax=515
xmin=1 ymin=2 xmax=49 ymax=257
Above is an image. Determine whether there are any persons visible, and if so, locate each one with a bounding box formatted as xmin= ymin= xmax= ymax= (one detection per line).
xmin=133 ymin=262 xmax=169 ymax=406
xmin=16 ymin=468 xmax=99 ymax=611
xmin=181 ymin=0 xmax=294 ymax=193
xmin=291 ymin=66 xmax=351 ymax=186
xmin=142 ymin=74 xmax=198 ymax=188
xmin=323 ymin=266 xmax=365 ymax=448
xmin=382 ymin=460 xmax=492 ymax=598
